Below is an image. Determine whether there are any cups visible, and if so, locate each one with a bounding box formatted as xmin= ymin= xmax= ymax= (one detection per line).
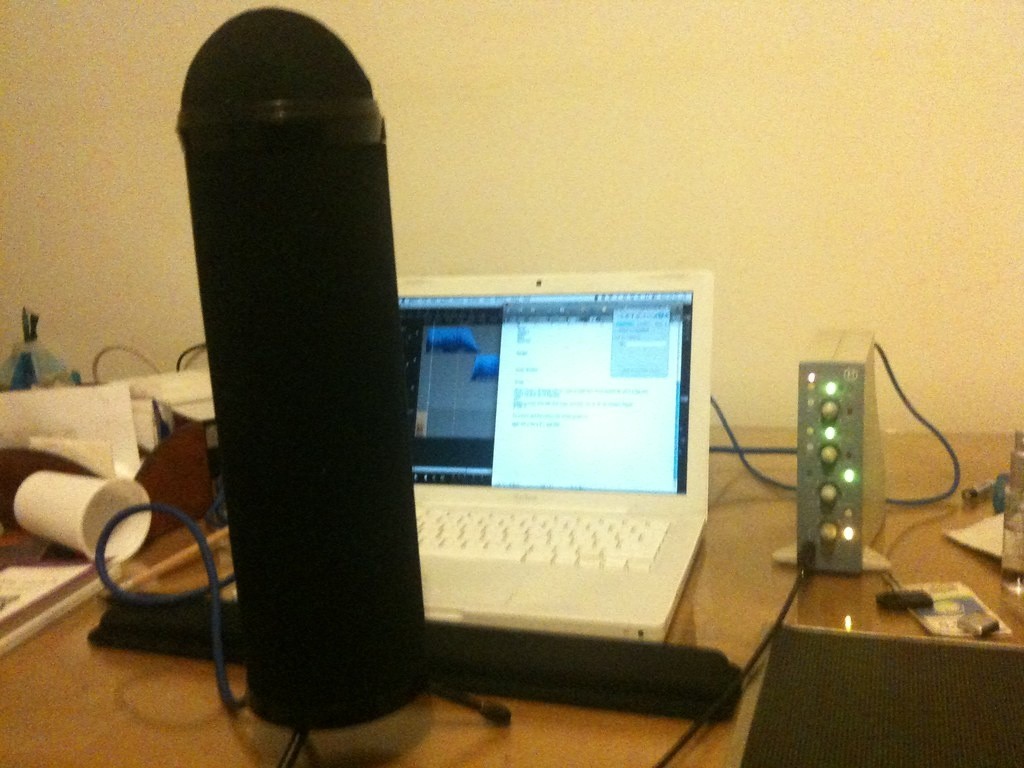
xmin=14 ymin=471 xmax=152 ymax=566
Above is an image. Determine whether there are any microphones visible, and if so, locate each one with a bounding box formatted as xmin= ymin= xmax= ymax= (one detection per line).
xmin=174 ymin=5 xmax=439 ymax=768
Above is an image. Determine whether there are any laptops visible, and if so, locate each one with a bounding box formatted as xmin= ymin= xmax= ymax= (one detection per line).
xmin=218 ymin=273 xmax=722 ymax=643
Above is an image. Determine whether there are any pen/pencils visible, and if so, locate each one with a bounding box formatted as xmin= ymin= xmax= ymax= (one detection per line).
xmin=21 ymin=305 xmax=31 ymax=342
xmin=101 ymin=526 xmax=231 ymax=599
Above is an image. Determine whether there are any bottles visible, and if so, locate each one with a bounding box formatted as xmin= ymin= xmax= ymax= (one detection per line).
xmin=1001 ymin=431 xmax=1024 ymax=596
